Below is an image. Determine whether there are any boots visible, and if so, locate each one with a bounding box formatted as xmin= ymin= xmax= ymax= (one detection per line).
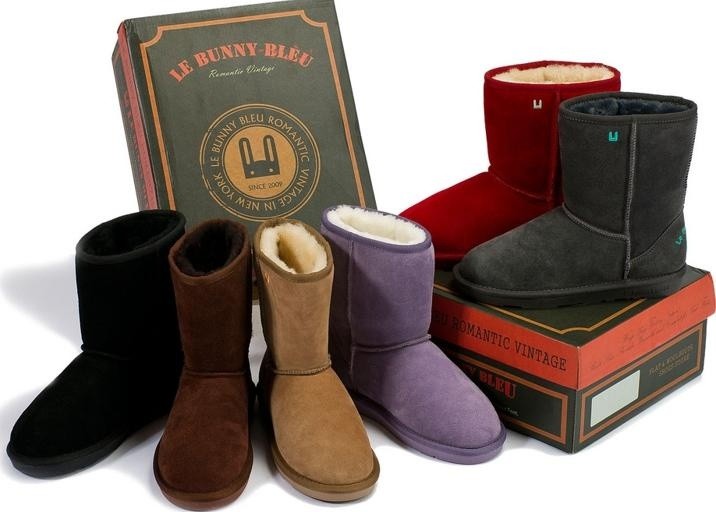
xmin=7 ymin=209 xmax=187 ymax=479
xmin=452 ymin=92 xmax=700 ymax=306
xmin=152 ymin=216 xmax=256 ymax=511
xmin=252 ymin=217 xmax=382 ymax=500
xmin=322 ymin=205 xmax=508 ymax=464
xmin=398 ymin=62 xmax=622 ymax=280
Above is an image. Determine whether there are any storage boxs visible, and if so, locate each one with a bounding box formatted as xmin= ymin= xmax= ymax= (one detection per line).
xmin=425 ymin=261 xmax=716 ymax=453
xmin=112 ymin=0 xmax=379 ymax=300
